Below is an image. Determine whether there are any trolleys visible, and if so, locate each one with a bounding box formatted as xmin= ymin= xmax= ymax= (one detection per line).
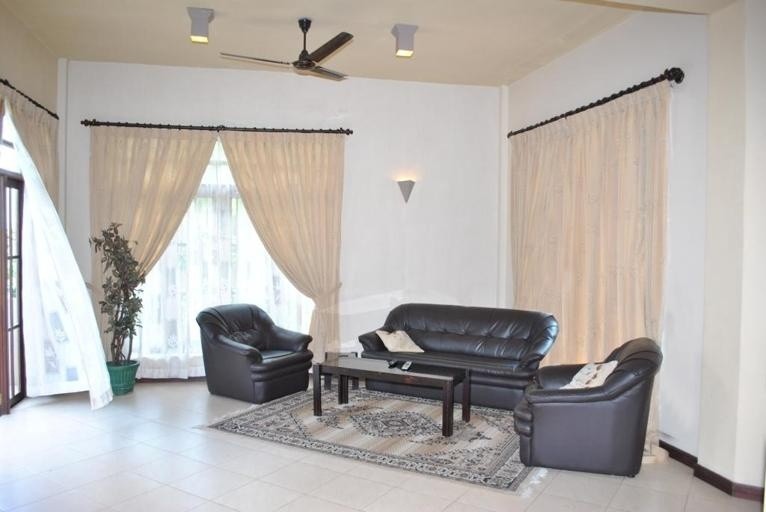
xmin=218 ymin=18 xmax=353 ymax=81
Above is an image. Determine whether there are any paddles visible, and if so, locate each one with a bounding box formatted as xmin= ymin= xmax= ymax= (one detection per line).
xmin=194 ymin=381 xmax=535 ymax=492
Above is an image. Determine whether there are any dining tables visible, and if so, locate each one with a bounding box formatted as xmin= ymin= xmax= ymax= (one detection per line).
xmin=87 ymin=221 xmax=147 ymax=396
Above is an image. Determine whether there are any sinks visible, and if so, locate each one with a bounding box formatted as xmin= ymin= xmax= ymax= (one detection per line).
xmin=513 ymin=337 xmax=663 ymax=477
xmin=358 ymin=304 xmax=559 ymax=410
xmin=196 ymin=304 xmax=313 ymax=404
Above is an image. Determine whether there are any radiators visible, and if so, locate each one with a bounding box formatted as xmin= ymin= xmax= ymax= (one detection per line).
xmin=401 ymin=360 xmax=413 ymax=370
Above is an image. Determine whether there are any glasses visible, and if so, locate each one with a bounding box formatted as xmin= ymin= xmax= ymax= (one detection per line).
xmin=397 ymin=180 xmax=416 ymax=203
xmin=187 ymin=7 xmax=215 ymax=44
xmin=391 ymin=24 xmax=418 ymax=58
